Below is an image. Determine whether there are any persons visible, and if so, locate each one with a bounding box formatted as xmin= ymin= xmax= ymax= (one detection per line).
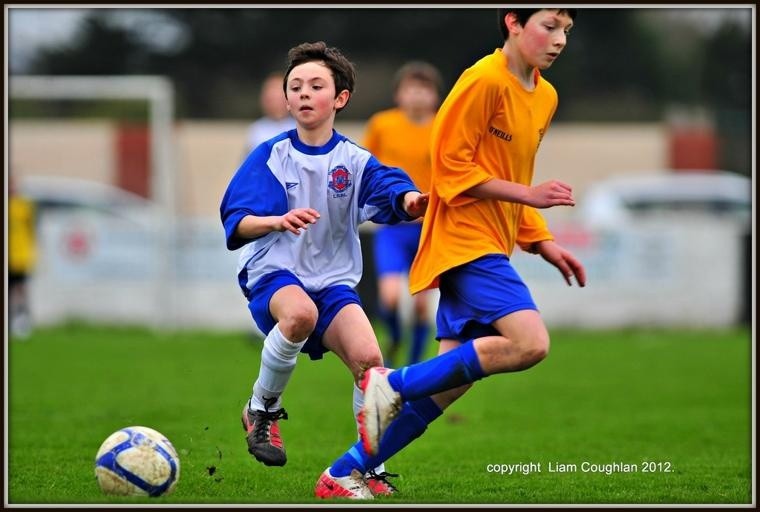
xmin=217 ymin=41 xmax=434 ymax=496
xmin=8 ymin=172 xmax=36 ymax=342
xmin=313 ymin=8 xmax=586 ymax=502
xmin=358 ymin=59 xmax=448 ymax=372
xmin=239 ymin=70 xmax=298 ymax=348
xmin=663 ymin=103 xmax=715 ymax=171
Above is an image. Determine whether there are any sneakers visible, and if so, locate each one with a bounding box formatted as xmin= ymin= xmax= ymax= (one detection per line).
xmin=242 ymin=398 xmax=287 ymax=465
xmin=366 ymin=472 xmax=398 ymax=496
xmin=315 ymin=467 xmax=375 ymax=499
xmin=357 ymin=367 xmax=402 ymax=457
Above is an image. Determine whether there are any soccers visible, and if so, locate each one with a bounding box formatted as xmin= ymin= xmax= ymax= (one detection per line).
xmin=94 ymin=425 xmax=181 ymax=498
